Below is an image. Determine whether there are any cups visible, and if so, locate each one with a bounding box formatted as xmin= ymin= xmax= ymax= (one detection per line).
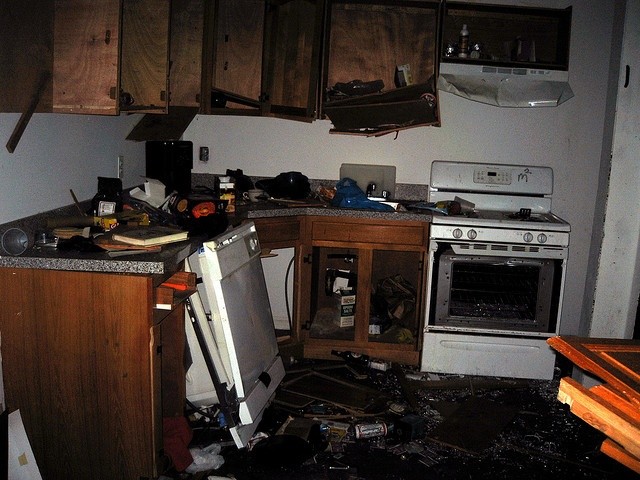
xmin=243 ymin=189 xmax=264 ymax=204
xmin=33 ymin=228 xmax=60 ymax=251
xmin=0 ymin=225 xmax=37 ymax=257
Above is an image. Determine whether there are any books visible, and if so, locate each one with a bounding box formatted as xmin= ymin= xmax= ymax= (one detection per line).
xmin=111 ymin=224 xmax=192 ymax=247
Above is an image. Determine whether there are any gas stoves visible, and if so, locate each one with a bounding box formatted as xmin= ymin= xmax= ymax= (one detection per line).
xmin=427 ymin=158 xmax=572 ymax=247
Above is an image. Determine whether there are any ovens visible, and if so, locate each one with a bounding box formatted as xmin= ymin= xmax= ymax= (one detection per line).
xmin=419 ymin=238 xmax=570 ymax=381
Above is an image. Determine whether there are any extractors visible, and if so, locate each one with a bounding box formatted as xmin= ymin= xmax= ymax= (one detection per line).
xmin=435 ymin=62 xmax=575 ymax=108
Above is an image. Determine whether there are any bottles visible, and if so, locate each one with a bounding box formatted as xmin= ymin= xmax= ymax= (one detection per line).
xmin=330 ymin=349 xmax=369 ymax=368
xmin=458 ymin=22 xmax=469 ymax=58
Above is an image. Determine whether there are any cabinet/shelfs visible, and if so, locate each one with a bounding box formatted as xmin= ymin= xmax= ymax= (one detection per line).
xmin=245 ymin=208 xmax=305 ymax=351
xmin=305 ymin=208 xmax=430 ymax=372
xmin=323 ymin=0 xmax=440 ymax=140
xmin=210 ymin=0 xmax=322 ymax=123
xmin=1 ymin=0 xmax=207 ymax=113
xmin=439 ymin=0 xmax=573 ymax=84
xmin=0 ymin=246 xmax=184 ymax=479
